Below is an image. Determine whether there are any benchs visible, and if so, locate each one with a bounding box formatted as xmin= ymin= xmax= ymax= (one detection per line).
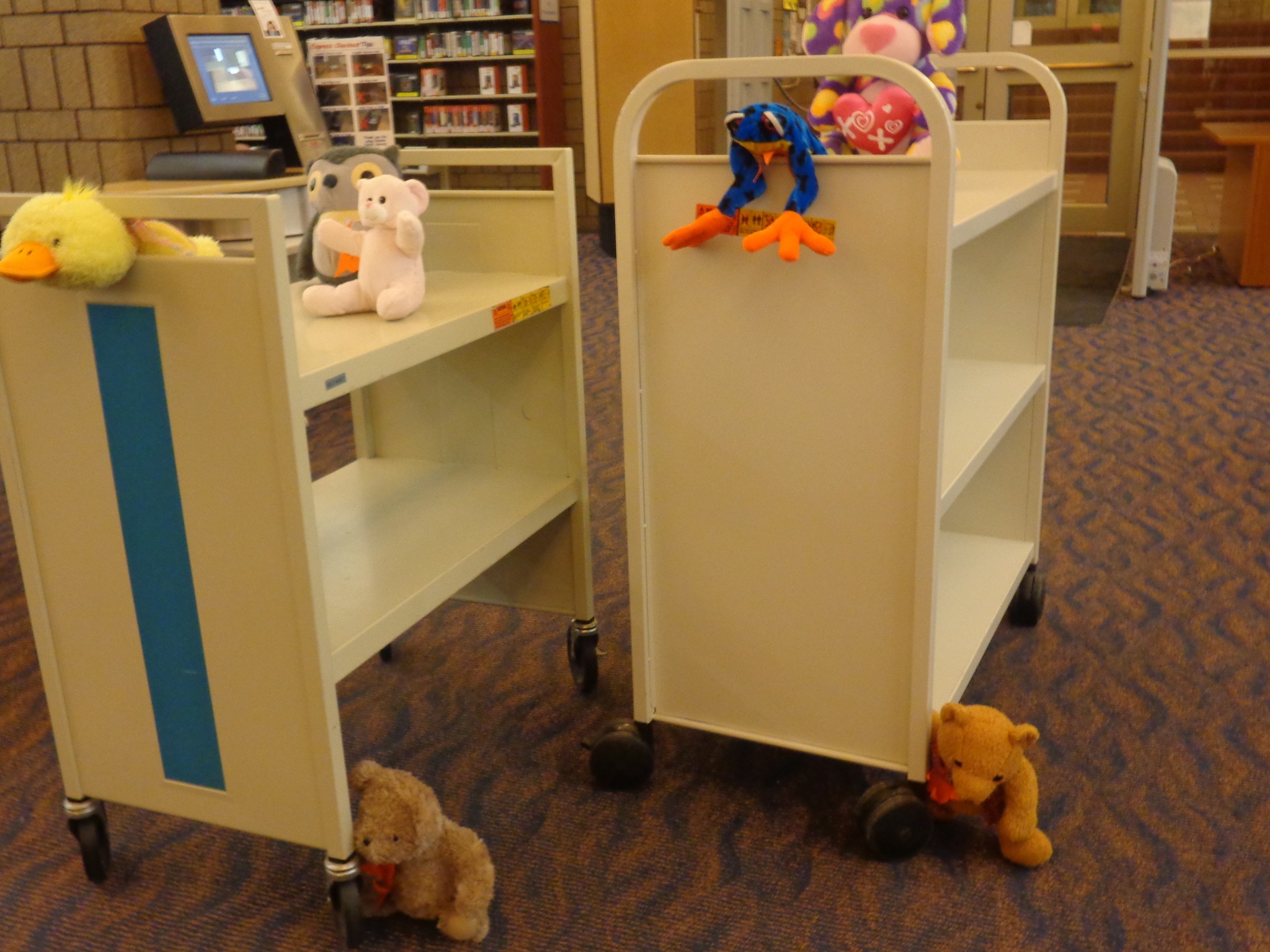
xmin=1197 ymin=120 xmax=1270 ymax=289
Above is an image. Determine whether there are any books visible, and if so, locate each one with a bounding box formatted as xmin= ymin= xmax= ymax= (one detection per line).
xmin=219 ymin=0 xmax=536 ymax=133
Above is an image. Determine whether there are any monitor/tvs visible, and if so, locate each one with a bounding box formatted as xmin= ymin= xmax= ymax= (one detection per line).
xmin=141 ymin=13 xmax=285 ymax=130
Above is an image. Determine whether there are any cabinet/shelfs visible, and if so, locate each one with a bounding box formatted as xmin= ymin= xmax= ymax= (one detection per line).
xmin=220 ymin=0 xmax=565 ymax=192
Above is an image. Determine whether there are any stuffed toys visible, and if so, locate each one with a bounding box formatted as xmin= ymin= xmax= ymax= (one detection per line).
xmin=298 ymin=146 xmax=402 ymax=286
xmin=662 ymin=101 xmax=834 ymax=261
xmin=348 ymin=759 xmax=495 ymax=942
xmin=800 ymin=0 xmax=967 ymax=161
xmin=0 ymin=177 xmax=224 ymax=290
xmin=302 ymin=175 xmax=429 ymax=321
xmin=928 ymin=702 xmax=1053 ymax=866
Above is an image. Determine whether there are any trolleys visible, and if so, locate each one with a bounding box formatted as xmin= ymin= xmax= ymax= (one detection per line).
xmin=587 ymin=50 xmax=1068 ymax=859
xmin=0 ymin=146 xmax=600 ymax=952
